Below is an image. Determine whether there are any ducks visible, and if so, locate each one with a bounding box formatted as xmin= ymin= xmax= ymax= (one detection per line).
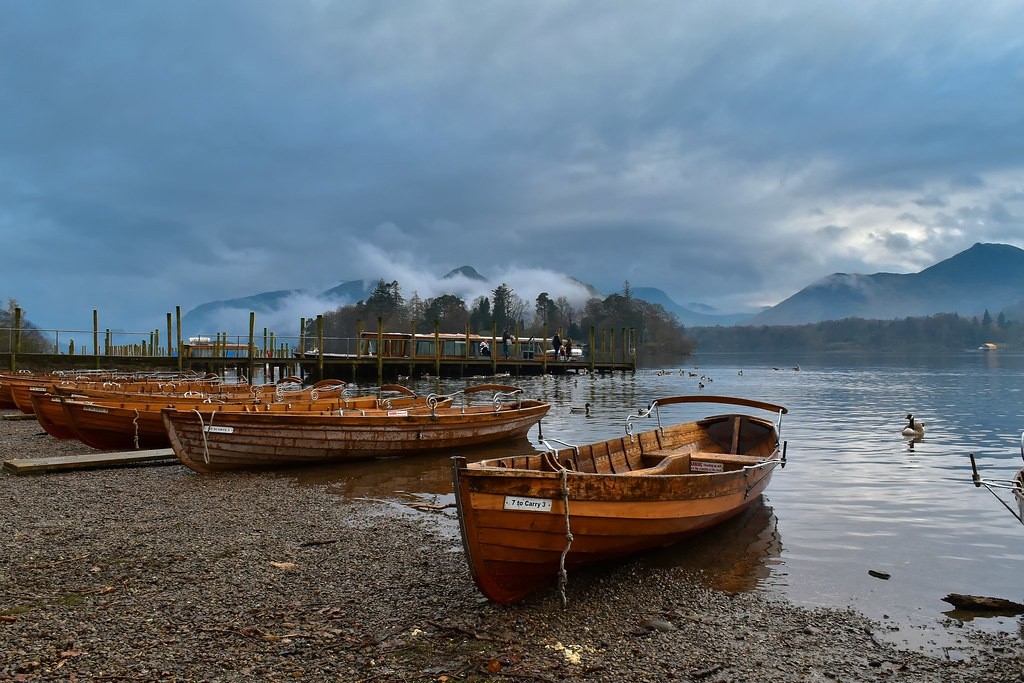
xmin=902 ymin=413 xmax=926 ymax=435
xmin=397 ymin=364 xmax=800 ymax=417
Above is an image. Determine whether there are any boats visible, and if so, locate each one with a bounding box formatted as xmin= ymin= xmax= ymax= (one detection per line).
xmin=181 ymin=343 xmax=258 ymax=357
xmin=0 ymin=370 xmax=552 ymax=473
xmin=977 ymin=343 xmax=997 ymax=352
xmin=292 ymin=303 xmax=582 ymax=379
xmin=448 ymin=394 xmax=787 ymax=607
xmin=939 ymin=431 xmax=1024 ymax=526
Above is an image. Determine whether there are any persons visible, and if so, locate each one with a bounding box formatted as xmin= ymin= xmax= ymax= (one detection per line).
xmin=502 ymin=327 xmax=511 ymax=360
xmin=480 ymin=339 xmax=492 ymax=357
xmin=552 ymin=332 xmax=564 ymax=362
xmin=565 ymin=338 xmax=572 ymax=363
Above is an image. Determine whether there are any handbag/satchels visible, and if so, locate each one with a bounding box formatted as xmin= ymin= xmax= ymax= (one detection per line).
xmin=506 ymin=339 xmax=512 ymax=345
